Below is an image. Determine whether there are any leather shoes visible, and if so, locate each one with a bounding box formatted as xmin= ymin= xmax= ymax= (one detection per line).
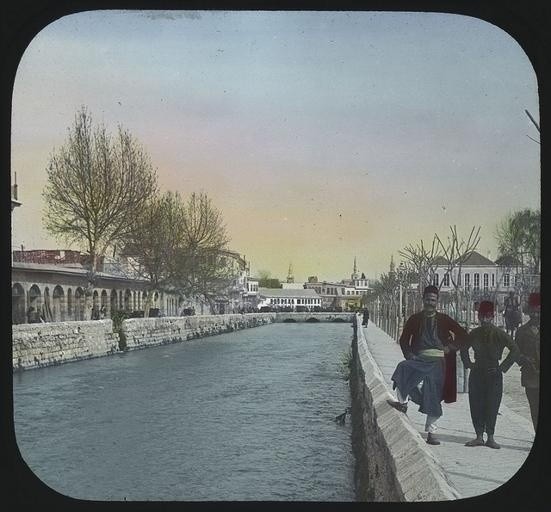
xmin=387 ymin=399 xmax=408 ymax=414
xmin=427 ymin=437 xmax=440 ymax=445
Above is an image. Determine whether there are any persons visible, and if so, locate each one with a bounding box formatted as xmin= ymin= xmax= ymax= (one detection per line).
xmin=387 ymin=285 xmax=540 ymax=449
xmin=362 ymin=308 xmax=369 ymax=328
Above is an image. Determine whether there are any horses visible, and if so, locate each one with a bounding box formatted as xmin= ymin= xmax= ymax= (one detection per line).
xmin=504 ymin=306 xmax=523 ymax=340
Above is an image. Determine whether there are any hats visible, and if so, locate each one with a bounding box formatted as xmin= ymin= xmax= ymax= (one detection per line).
xmin=425 ymin=286 xmax=438 ymax=293
xmin=480 ymin=301 xmax=493 ymax=311
xmin=529 ymin=293 xmax=541 ymax=305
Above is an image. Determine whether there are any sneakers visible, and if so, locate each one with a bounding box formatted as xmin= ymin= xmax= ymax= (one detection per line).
xmin=466 ymin=439 xmax=484 ymax=446
xmin=485 ymin=441 xmax=500 ymax=449
